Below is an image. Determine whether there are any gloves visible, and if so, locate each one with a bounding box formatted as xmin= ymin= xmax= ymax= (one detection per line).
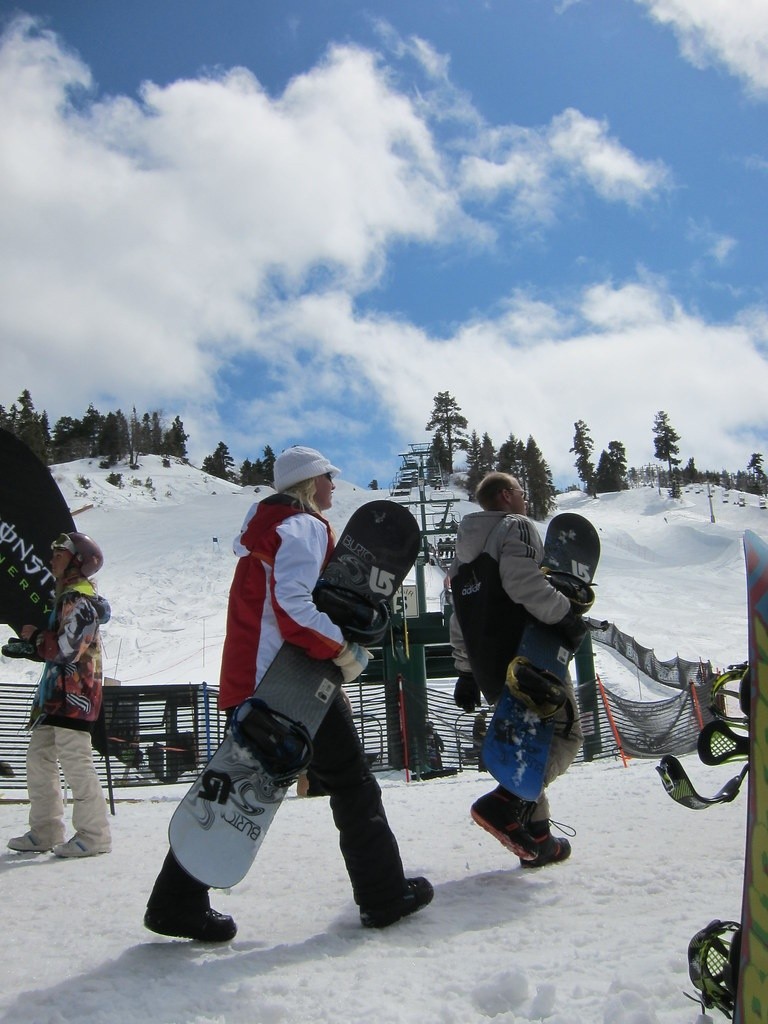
xmin=2 ymin=637 xmax=36 ymax=659
xmin=454 ymin=671 xmax=482 ymax=714
xmin=549 ymin=608 xmax=588 ymax=651
xmin=333 ymin=642 xmax=375 ymax=683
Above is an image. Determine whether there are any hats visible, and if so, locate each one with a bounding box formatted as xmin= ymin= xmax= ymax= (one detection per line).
xmin=273 ymin=446 xmax=341 ymax=494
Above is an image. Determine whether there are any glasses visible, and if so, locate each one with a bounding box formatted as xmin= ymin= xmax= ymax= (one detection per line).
xmin=506 ymin=488 xmax=528 ymax=501
xmin=50 ymin=533 xmax=83 ymax=562
xmin=325 ymin=472 xmax=334 ymax=481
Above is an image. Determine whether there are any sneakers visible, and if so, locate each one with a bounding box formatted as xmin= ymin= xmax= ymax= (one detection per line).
xmin=54 ymin=831 xmax=113 ymax=857
xmin=145 ymin=907 xmax=238 ymax=942
xmin=359 ymin=876 xmax=434 ymax=928
xmin=470 ymin=784 xmax=540 ymax=861
xmin=7 ymin=832 xmax=48 ymax=852
xmin=519 ymin=819 xmax=577 ymax=869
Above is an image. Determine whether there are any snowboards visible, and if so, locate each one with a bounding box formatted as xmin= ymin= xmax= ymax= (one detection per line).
xmin=481 ymin=513 xmax=601 ymax=802
xmin=731 ymin=525 xmax=767 ymax=1024
xmin=165 ymin=498 xmax=422 ymax=890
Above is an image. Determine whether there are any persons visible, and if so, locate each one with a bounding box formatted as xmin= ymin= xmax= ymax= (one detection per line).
xmin=449 ymin=472 xmax=589 ymax=869
xmin=143 ymin=446 xmax=433 ymax=942
xmin=1 ymin=532 xmax=112 ymax=857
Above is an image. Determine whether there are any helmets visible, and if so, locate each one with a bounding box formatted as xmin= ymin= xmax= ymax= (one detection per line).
xmin=63 ymin=531 xmax=104 ymax=579
